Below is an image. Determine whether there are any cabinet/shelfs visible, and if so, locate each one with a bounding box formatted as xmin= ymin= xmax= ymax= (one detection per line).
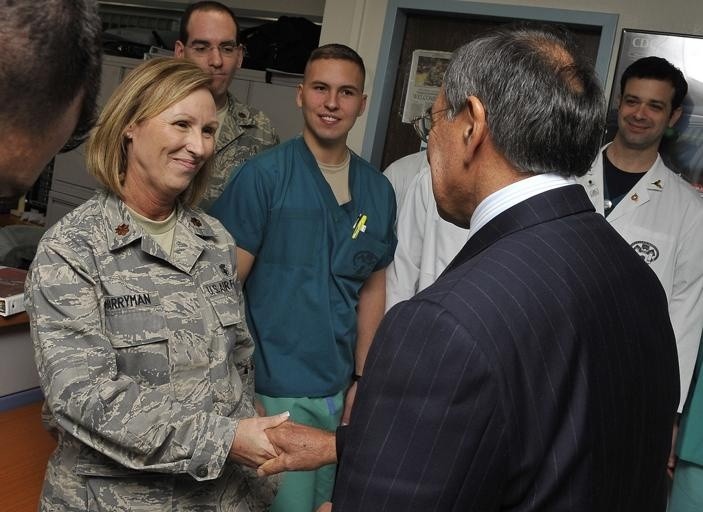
xmin=45 ymin=54 xmax=305 ymax=232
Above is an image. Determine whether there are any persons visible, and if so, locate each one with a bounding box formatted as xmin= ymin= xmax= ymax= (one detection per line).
xmin=260 ymin=30 xmax=677 ymax=511
xmin=371 ymin=142 xmax=481 ymax=325
xmin=159 ymin=1 xmax=280 ymax=226
xmin=0 ymin=0 xmax=101 ymax=212
xmin=27 ymin=58 xmax=290 ymax=512
xmin=208 ymin=42 xmax=397 ymax=511
xmin=573 ymin=54 xmax=702 ymax=494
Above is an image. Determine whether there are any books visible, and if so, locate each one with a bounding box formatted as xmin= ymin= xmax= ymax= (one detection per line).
xmin=0 ymin=265 xmax=29 ymax=323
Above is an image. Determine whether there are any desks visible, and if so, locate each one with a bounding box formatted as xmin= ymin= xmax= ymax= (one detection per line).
xmin=1 ymin=265 xmax=59 ymax=511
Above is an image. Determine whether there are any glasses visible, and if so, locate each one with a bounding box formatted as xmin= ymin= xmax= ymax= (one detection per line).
xmin=411 ymin=108 xmax=453 ymax=143
xmin=185 ymin=44 xmax=238 ymax=57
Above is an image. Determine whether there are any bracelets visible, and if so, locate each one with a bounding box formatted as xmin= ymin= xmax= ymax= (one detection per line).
xmin=352 ymin=371 xmax=362 ymax=383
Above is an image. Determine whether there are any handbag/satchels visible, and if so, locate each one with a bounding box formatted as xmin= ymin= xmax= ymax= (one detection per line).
xmin=236 ymin=15 xmax=321 ymax=73
